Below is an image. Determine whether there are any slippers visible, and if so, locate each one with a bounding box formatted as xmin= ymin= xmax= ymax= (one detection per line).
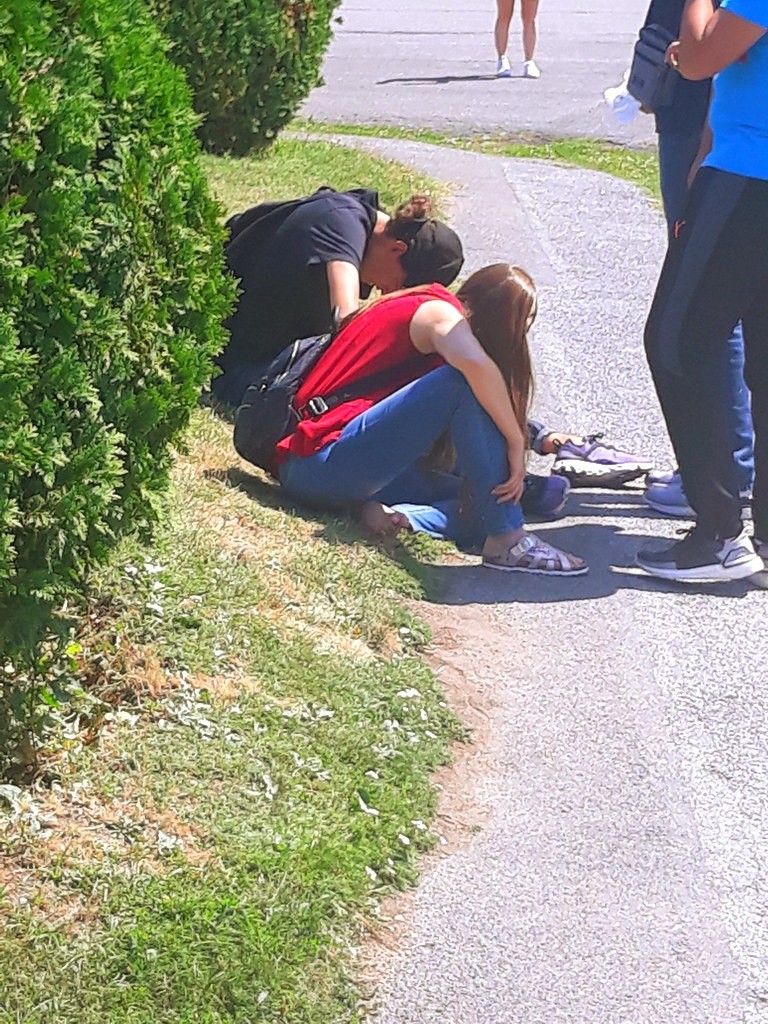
xmin=479 ymin=530 xmax=591 ymax=578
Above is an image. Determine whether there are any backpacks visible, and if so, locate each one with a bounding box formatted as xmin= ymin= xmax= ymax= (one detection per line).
xmin=230 ymin=304 xmax=452 ymax=473
xmin=212 ymin=183 xmax=351 ymax=280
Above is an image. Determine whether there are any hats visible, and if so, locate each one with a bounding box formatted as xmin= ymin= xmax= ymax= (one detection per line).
xmin=399 ymin=215 xmax=467 ymax=291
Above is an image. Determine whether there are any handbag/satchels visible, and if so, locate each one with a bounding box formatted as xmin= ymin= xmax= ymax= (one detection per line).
xmin=626 ymin=23 xmax=682 ymax=114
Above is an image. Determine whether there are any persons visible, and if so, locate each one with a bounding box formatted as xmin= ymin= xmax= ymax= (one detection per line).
xmin=494 ymin=0 xmax=542 ymax=78
xmin=273 ymin=260 xmax=590 ymax=577
xmin=220 ymin=187 xmax=652 ymax=514
xmin=630 ymin=1 xmax=766 ymax=583
xmin=639 ymin=0 xmax=756 ymax=523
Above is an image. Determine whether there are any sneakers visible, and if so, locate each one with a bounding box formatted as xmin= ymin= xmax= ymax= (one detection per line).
xmin=734 ymin=534 xmax=768 ymax=589
xmin=634 ymin=526 xmax=764 ymax=583
xmin=643 ymin=479 xmax=752 ymax=519
xmin=497 ymin=55 xmax=511 ymax=79
xmin=645 ymin=466 xmax=685 ymax=485
xmin=551 ymin=433 xmax=657 ymax=488
xmin=523 ymin=60 xmax=540 ymax=79
xmin=517 ymin=471 xmax=572 ymax=517
